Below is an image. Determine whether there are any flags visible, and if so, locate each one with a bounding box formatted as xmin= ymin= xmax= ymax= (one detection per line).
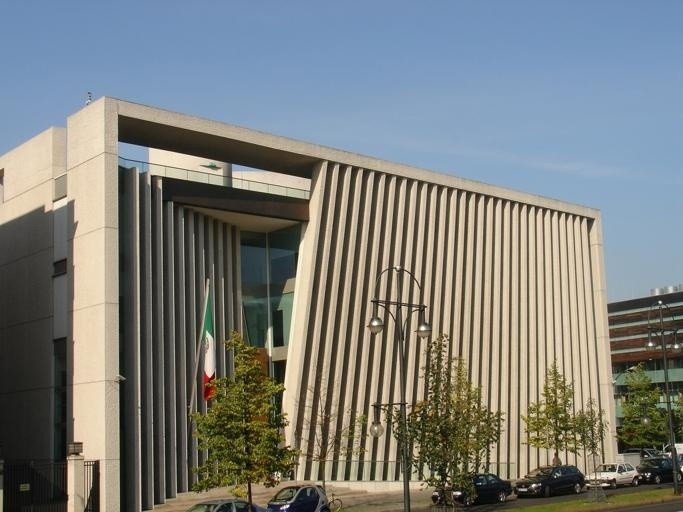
xmin=195 ymin=286 xmax=218 ymax=401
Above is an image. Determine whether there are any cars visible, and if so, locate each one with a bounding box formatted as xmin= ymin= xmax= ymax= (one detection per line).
xmin=429 ymin=456 xmax=682 ymax=504
xmin=186 ymin=500 xmax=266 ymax=512
xmin=265 ymin=483 xmax=327 ymax=512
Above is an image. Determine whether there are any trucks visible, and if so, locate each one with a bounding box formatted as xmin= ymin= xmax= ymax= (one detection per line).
xmin=658 ymin=442 xmax=682 ymax=456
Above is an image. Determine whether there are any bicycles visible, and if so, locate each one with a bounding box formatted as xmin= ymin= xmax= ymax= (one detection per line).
xmin=325 ymin=490 xmax=342 ymax=512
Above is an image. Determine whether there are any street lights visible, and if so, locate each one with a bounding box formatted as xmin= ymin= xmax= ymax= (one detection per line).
xmin=646 ymin=299 xmax=682 ymax=494
xmin=360 ymin=265 xmax=431 ymax=512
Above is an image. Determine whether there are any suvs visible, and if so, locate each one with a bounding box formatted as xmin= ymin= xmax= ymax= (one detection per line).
xmin=621 ymin=447 xmax=665 ymax=457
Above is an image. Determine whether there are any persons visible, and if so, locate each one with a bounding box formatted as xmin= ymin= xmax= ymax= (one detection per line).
xmin=553 ymin=453 xmax=562 ymax=467
